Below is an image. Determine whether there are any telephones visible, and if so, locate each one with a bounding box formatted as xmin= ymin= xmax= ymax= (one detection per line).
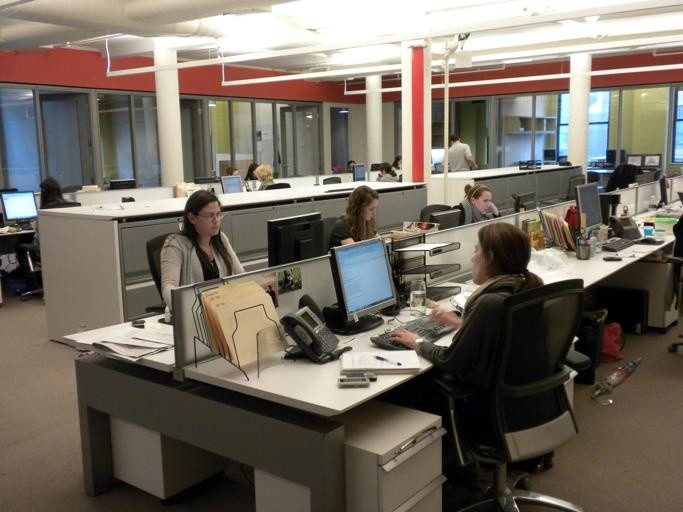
xmin=609 ymin=216 xmax=641 ymax=239
xmin=279 ymin=306 xmax=339 ymax=363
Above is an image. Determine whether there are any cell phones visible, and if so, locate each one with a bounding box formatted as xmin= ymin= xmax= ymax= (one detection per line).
xmin=337 ymin=377 xmax=369 ymax=387
xmin=603 ymin=257 xmax=621 ymax=261
xmin=347 ymin=372 xmax=377 ymax=380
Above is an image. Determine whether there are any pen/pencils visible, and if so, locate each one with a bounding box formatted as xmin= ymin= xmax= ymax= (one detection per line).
xmin=616 ymin=254 xmax=635 ymax=257
xmin=375 ymin=356 xmax=402 ymax=365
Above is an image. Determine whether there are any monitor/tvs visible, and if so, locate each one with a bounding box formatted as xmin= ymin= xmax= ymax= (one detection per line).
xmin=435 ymin=163 xmax=451 ymax=174
xmin=110 ymin=179 xmax=136 ymax=190
xmin=322 ymin=236 xmax=397 ymax=335
xmin=606 ymin=149 xmax=660 ymax=167
xmin=0 ymin=191 xmax=38 ymax=224
xmin=575 ymin=182 xmax=603 ymax=245
xmin=656 ymin=175 xmax=667 ymax=210
xmin=429 ymin=209 xmax=460 ymax=231
xmin=221 ymin=176 xmax=243 ymax=194
xmin=514 ymin=192 xmax=537 ymax=213
xmin=267 ymin=211 xmax=322 ymax=267
xmin=353 ymin=164 xmax=365 ymax=182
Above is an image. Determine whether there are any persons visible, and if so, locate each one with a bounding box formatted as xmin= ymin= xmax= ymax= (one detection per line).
xmin=253 ymin=164 xmax=275 ymax=191
xmin=451 ymin=185 xmax=502 ymax=227
xmin=15 ymin=178 xmax=67 ymax=294
xmin=346 ymin=160 xmax=359 ymax=173
xmin=387 ymin=223 xmax=546 ymax=450
xmin=160 ymin=190 xmax=246 ymax=312
xmin=376 ymin=162 xmax=398 ymax=182
xmin=442 ymin=134 xmax=478 ymax=172
xmin=245 ymin=164 xmax=260 ymax=181
xmin=326 ymin=186 xmax=379 ymax=252
xmin=391 ymin=156 xmax=402 ymax=181
xmin=226 ymin=167 xmax=241 ymax=177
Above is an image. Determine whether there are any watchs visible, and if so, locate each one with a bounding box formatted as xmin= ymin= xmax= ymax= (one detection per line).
xmin=415 ymin=336 xmax=426 ymax=348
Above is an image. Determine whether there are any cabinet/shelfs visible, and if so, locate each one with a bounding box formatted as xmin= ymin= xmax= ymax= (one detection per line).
xmin=432 ymin=120 xmax=443 ymax=137
xmin=503 ymin=115 xmax=559 ymax=136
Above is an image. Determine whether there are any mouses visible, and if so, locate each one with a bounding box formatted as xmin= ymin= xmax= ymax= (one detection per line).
xmin=641 ymin=238 xmax=657 ymax=243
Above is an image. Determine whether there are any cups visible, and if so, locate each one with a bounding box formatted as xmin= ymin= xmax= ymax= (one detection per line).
xmin=576 ymin=224 xmax=608 ymax=260
xmin=410 ymin=279 xmax=427 ymax=317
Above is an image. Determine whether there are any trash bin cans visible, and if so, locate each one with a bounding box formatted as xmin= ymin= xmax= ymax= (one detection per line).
xmin=575 ymin=309 xmax=608 ymax=385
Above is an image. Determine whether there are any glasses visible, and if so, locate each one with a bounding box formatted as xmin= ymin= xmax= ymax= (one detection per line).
xmin=198 ymin=210 xmax=225 ymax=221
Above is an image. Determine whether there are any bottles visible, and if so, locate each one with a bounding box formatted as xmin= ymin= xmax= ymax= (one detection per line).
xmin=648 ymin=195 xmax=657 ymax=214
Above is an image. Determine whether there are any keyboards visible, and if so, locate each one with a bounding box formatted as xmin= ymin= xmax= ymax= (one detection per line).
xmin=370 ymin=315 xmax=456 ymax=350
xmin=602 ymin=238 xmax=633 ymax=252
xmin=20 ymin=224 xmax=33 ymax=230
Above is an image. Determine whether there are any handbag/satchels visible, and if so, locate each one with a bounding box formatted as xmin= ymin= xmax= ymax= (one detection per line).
xmin=595 ymin=322 xmax=626 ymax=363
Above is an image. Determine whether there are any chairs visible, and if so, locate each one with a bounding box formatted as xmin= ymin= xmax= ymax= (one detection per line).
xmin=0 ymin=152 xmax=682 ymax=511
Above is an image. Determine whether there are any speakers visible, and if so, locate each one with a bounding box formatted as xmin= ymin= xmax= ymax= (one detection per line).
xmin=299 ymin=295 xmax=327 ymax=326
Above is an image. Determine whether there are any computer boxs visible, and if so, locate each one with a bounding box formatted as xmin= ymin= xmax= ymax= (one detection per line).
xmin=595 ymin=286 xmax=649 ymax=335
